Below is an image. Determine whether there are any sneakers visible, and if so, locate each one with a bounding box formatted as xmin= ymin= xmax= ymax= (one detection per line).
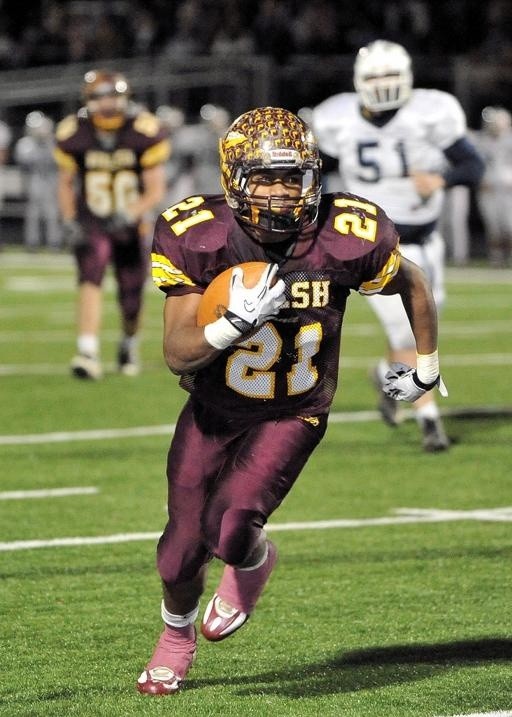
xmin=414 ymin=405 xmax=449 ymax=450
xmin=373 ymin=361 xmax=398 ymax=426
xmin=199 ymin=539 xmax=279 ymax=642
xmin=137 ymin=625 xmax=197 ymax=696
xmin=118 ymin=332 xmax=138 ymax=376
xmin=71 ymin=351 xmax=100 ymax=380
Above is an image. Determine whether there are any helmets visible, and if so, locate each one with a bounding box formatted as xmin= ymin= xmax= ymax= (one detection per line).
xmin=218 ymin=106 xmax=322 ymax=234
xmin=81 ymin=69 xmax=132 ymax=132
xmin=352 ymin=38 xmax=415 ymax=116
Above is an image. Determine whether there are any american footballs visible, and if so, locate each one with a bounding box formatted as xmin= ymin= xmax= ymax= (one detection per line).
xmin=196 ymin=261 xmax=278 ymax=326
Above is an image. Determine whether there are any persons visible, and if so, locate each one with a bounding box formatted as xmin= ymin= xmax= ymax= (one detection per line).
xmin=134 ymin=106 xmax=447 ymax=694
xmin=310 ymin=40 xmax=485 ymax=450
xmin=53 ymin=67 xmax=170 ymax=378
xmin=1 ymin=2 xmax=512 ymax=268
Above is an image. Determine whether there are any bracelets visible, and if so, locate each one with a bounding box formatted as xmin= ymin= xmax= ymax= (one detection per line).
xmin=415 ymin=348 xmax=439 ymax=383
xmin=203 ymin=317 xmax=242 ymax=349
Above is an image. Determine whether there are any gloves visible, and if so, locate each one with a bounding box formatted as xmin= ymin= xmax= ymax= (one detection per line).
xmin=382 ymin=348 xmax=450 ymax=404
xmin=104 ymin=210 xmax=133 ymax=235
xmin=61 ymin=220 xmax=95 ymax=258
xmin=203 ymin=261 xmax=287 ymax=353
